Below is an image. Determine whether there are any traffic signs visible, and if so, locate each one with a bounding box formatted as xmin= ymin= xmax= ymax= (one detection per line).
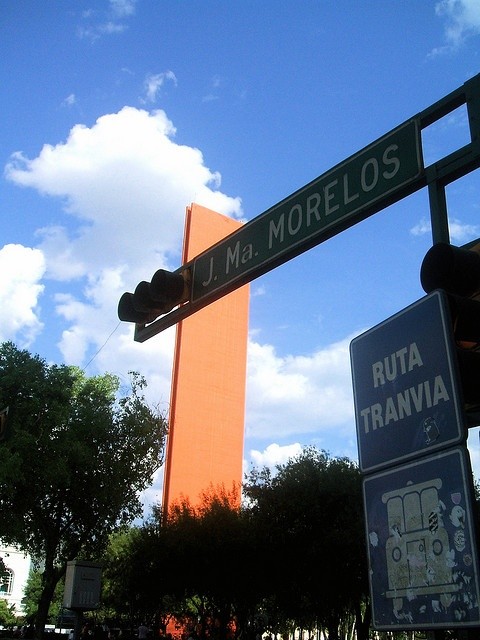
xmin=191 ymin=116 xmax=424 ymax=304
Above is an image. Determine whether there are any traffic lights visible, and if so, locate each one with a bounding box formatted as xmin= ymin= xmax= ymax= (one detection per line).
xmin=419 ymin=239 xmax=478 ymax=428
xmin=118 ymin=269 xmax=183 ymax=324
